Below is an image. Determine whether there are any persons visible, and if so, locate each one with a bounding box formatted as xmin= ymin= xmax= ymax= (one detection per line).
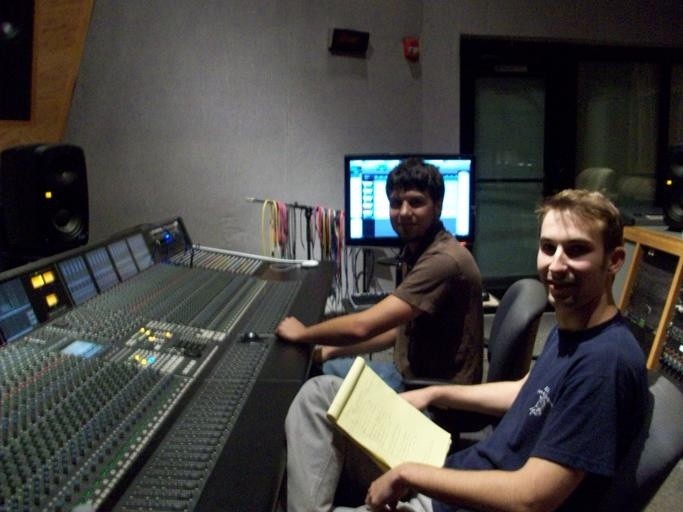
xmin=276 ymin=155 xmax=484 ymax=512
xmin=339 ymin=188 xmax=649 ymax=512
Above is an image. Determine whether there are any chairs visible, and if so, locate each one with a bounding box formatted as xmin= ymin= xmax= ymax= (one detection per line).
xmin=559 ymin=376 xmax=682 ymax=512
xmin=399 ymin=278 xmax=548 ymax=442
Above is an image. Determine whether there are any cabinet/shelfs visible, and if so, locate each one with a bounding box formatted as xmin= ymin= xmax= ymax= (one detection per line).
xmin=612 ymin=226 xmax=681 ymax=375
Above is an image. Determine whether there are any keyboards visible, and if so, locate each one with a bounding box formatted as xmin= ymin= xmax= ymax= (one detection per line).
xmin=348 ymin=291 xmax=395 ymax=309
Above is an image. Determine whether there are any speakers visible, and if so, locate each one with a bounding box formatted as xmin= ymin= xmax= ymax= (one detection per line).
xmin=0 ymin=0 xmax=36 ymax=121
xmin=662 ymin=144 xmax=683 ymax=229
xmin=0 ymin=141 xmax=89 ymax=272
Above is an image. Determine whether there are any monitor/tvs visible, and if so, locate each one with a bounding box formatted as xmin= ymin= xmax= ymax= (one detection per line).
xmin=344 ymin=153 xmax=477 ymax=265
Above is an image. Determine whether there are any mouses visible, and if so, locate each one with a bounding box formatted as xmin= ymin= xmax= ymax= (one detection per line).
xmin=481 ymin=287 xmax=489 ymax=301
